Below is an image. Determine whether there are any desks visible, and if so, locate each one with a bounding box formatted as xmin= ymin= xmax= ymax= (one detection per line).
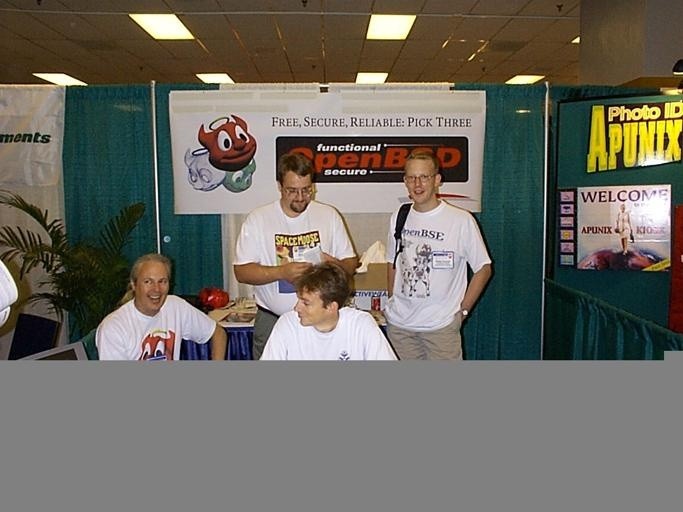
xmin=187 ymin=300 xmax=388 ymax=361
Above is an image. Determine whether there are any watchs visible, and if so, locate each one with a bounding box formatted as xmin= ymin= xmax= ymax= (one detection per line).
xmin=461 ymin=308 xmax=470 ymax=318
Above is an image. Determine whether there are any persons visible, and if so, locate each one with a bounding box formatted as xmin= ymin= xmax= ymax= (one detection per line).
xmin=614 ymin=204 xmax=633 ymax=257
xmin=233 ymin=154 xmax=400 ymax=361
xmin=382 ymin=147 xmax=492 ymax=360
xmin=96 ymin=253 xmax=228 ymax=361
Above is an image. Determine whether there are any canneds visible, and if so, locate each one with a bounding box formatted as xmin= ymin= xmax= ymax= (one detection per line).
xmin=371 ymin=295 xmax=380 ymax=311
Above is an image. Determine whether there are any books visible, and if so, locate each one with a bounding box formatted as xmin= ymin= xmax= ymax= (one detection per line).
xmin=217 ymin=308 xmax=259 ymax=329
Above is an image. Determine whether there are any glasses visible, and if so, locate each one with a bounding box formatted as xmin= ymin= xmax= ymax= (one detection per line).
xmin=405 ymin=173 xmax=438 ymax=184
xmin=280 ymin=183 xmax=315 ymax=195
